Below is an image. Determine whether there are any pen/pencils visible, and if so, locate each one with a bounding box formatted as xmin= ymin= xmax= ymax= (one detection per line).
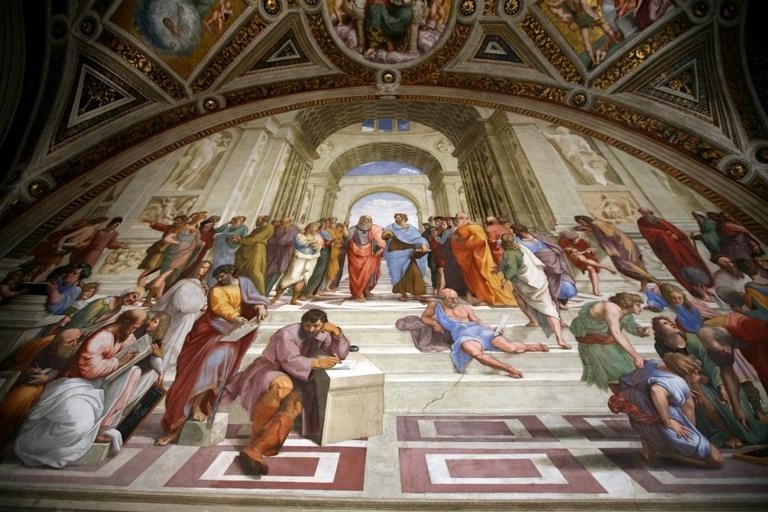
xmin=334 ymin=353 xmax=342 ymax=364
xmin=35 ymin=361 xmax=42 ymax=374
